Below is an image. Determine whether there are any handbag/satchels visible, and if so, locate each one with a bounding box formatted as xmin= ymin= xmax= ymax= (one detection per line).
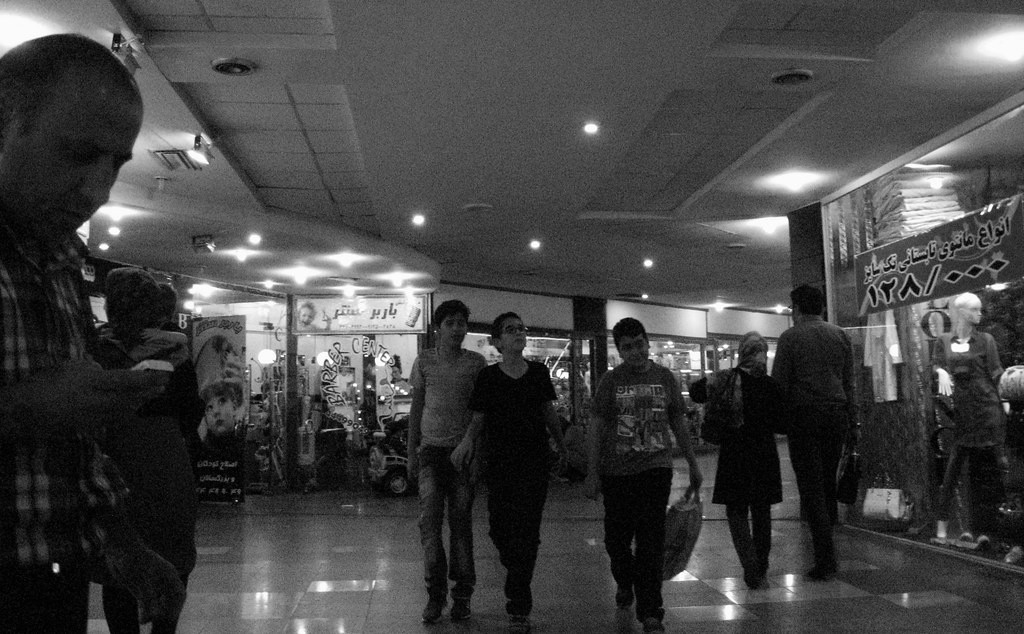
xmin=662 ymin=485 xmax=702 ymax=580
xmin=700 ymin=370 xmax=742 ymax=443
xmin=836 ymin=440 xmax=863 ymax=504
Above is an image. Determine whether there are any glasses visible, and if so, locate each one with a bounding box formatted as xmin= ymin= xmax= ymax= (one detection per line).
xmin=501 ymin=325 xmax=528 ymax=335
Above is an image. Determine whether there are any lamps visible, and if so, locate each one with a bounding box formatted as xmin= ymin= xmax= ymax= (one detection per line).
xmin=208 ymin=233 xmax=232 ymax=259
xmin=110 ymin=32 xmax=141 ymax=76
xmin=188 ymin=136 xmax=215 ymax=166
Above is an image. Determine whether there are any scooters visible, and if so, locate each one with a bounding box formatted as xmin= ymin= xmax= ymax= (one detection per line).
xmin=366 ymin=410 xmax=412 ymax=494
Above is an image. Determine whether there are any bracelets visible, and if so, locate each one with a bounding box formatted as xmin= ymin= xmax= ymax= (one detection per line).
xmin=852 ymin=423 xmax=862 ymax=427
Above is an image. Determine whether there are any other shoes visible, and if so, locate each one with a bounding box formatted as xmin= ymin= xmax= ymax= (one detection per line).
xmin=421 ymin=597 xmax=447 ymax=622
xmin=616 ymin=586 xmax=634 ymax=608
xmin=506 ymin=601 xmax=529 ymax=620
xmin=643 ymin=615 xmax=664 ymax=631
xmin=450 ymin=598 xmax=471 ymax=619
xmin=807 ymin=570 xmax=832 ymax=581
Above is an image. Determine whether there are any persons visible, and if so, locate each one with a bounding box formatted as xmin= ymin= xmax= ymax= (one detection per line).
xmin=585 ymin=317 xmax=702 ymax=634
xmin=390 ymin=354 xmax=407 ymax=383
xmin=690 ymin=333 xmax=786 ymax=589
xmin=405 ymin=298 xmax=487 ymax=622
xmin=297 ymin=302 xmax=332 ymax=333
xmin=0 ymin=32 xmax=188 ymax=634
xmin=557 ymin=404 xmax=572 ymax=425
xmin=199 ymin=369 xmax=242 ymax=455
xmin=95 ymin=267 xmax=198 ymax=634
xmin=929 ymin=291 xmax=1024 ymax=564
xmin=450 ymin=311 xmax=568 ymax=633
xmin=771 ymin=284 xmax=863 ymax=577
